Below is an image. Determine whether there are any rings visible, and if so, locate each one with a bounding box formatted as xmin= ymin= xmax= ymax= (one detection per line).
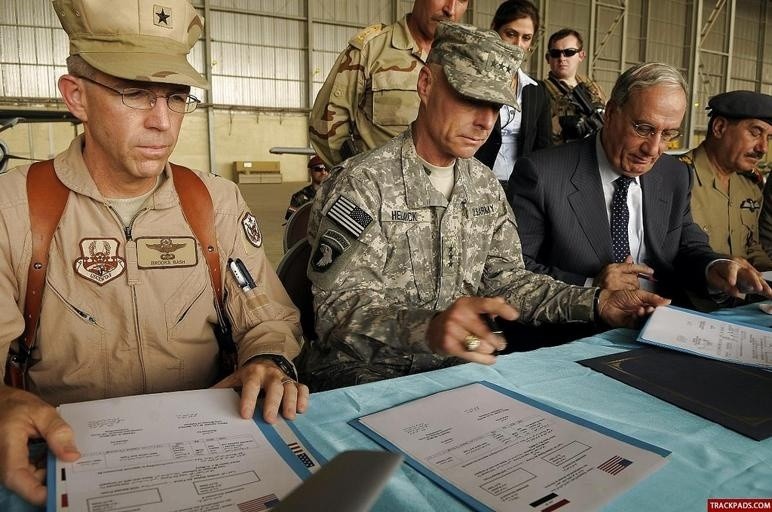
xmin=464 ymin=335 xmax=481 ymax=351
xmin=282 ymin=378 xmax=295 ymax=385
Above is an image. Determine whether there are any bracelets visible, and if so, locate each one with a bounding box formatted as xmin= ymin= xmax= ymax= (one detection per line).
xmin=593 ymin=288 xmax=603 ymax=319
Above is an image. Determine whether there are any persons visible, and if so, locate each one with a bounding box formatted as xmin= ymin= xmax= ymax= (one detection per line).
xmin=759 ymin=170 xmax=772 ymax=261
xmin=305 ymin=19 xmax=673 ymax=388
xmin=309 ymin=0 xmax=468 ymax=170
xmin=737 ymin=167 xmax=764 ymax=192
xmin=540 ymin=27 xmax=608 ymax=148
xmin=475 ymin=1 xmax=553 ymax=199
xmin=285 ymin=156 xmax=331 ymax=222
xmin=0 ymin=0 xmax=309 ymax=506
xmin=674 ymin=90 xmax=771 ymax=314
xmin=507 ymin=61 xmax=772 ymax=360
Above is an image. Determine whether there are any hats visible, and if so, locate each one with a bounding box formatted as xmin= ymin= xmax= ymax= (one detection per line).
xmin=705 ymin=89 xmax=772 ymax=125
xmin=54 ymin=0 xmax=215 ymax=93
xmin=307 ymin=156 xmax=325 ymax=168
xmin=426 ymin=21 xmax=526 ymax=115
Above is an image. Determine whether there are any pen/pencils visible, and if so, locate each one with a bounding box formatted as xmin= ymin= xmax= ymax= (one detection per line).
xmin=227 ymin=258 xmax=257 ymax=294
xmin=636 ymin=273 xmax=658 ymax=282
xmin=463 ymin=280 xmax=504 ymax=337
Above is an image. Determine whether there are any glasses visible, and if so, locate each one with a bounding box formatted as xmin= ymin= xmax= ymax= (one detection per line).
xmin=312 ymin=167 xmax=328 ymax=171
xmin=620 ymin=107 xmax=681 ymax=144
xmin=78 ymin=75 xmax=201 ymax=114
xmin=548 ymin=47 xmax=580 ymax=60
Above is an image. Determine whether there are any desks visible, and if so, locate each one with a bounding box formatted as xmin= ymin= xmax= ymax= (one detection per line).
xmin=0 ymin=278 xmax=772 ymax=512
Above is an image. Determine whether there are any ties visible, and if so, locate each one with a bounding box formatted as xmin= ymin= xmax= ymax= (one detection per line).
xmin=609 ymin=177 xmax=635 ymax=263
